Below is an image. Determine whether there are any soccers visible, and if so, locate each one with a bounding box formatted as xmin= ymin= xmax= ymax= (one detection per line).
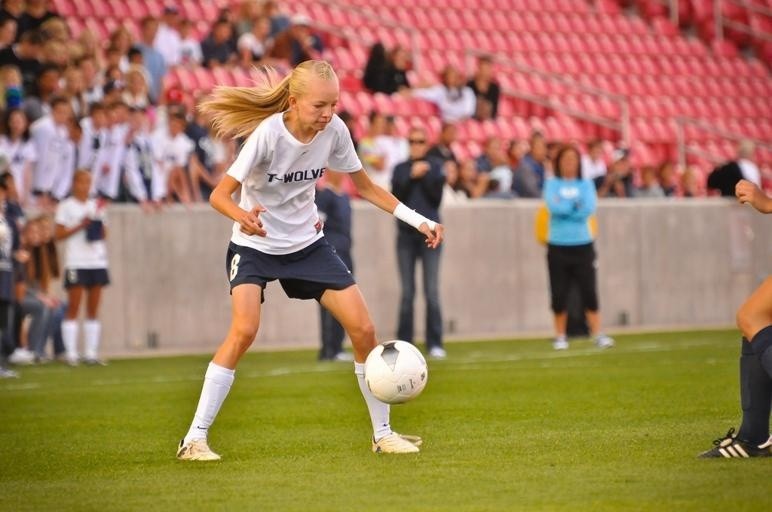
xmin=364 ymin=340 xmax=428 ymax=403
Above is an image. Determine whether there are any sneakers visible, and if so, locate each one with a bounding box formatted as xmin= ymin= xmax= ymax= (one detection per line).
xmin=696 ymin=430 xmax=771 ymax=460
xmin=430 ymin=345 xmax=448 ymax=361
xmin=591 ymin=331 xmax=617 ymax=351
xmin=553 ymin=335 xmax=570 ymax=351
xmin=370 ymin=431 xmax=422 ymax=455
xmin=176 ymin=436 xmax=222 ymax=462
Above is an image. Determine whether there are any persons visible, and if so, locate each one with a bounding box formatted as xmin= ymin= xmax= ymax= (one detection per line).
xmin=698 ymin=179 xmax=772 ymax=457
xmin=0 ymin=0 xmax=446 ymax=368
xmin=177 ymin=60 xmax=443 ymax=461
xmin=706 ymin=159 xmax=762 ymax=197
xmin=402 ymin=55 xmax=692 ymax=201
xmin=541 ymin=146 xmax=616 ymax=349
